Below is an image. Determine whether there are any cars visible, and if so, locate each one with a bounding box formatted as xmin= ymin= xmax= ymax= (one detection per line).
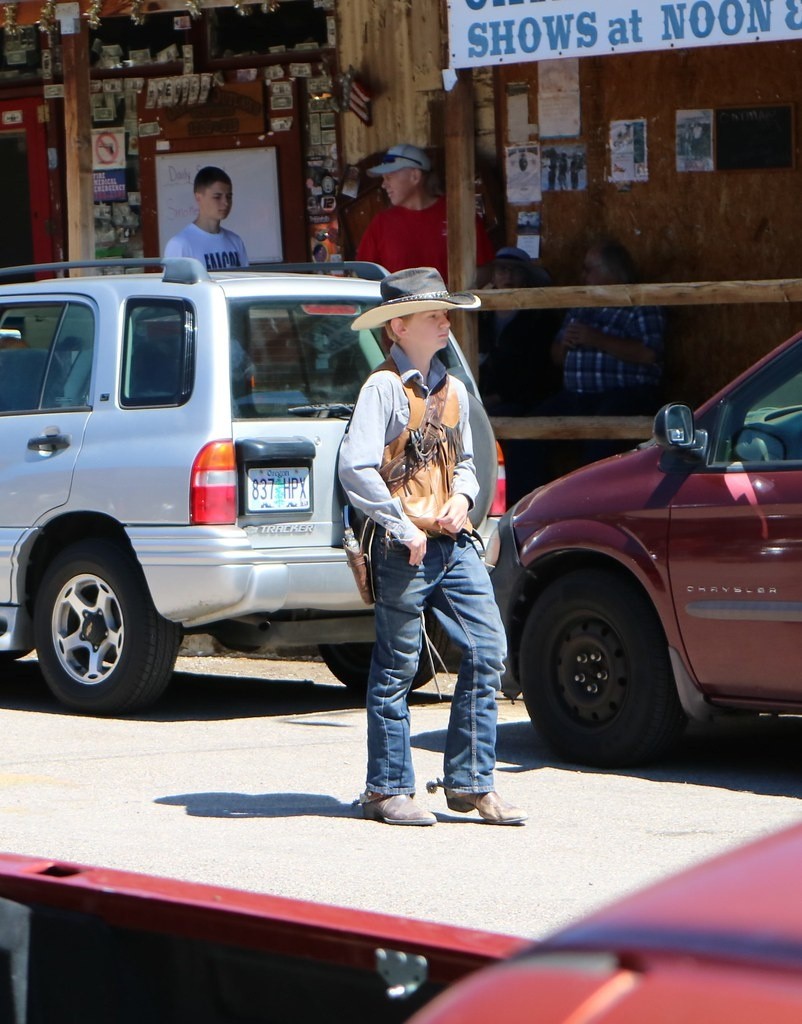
xmin=486 ymin=327 xmax=802 ymax=771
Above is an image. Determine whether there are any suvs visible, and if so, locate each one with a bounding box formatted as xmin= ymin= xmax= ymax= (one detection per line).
xmin=1 ymin=259 xmax=509 ymax=712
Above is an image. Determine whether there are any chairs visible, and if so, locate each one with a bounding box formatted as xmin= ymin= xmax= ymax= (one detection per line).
xmin=36 ymin=338 xmax=89 ymax=409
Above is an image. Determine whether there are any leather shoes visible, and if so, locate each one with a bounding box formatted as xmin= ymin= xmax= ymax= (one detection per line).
xmin=447 ymin=785 xmax=529 ymax=823
xmin=363 ymin=785 xmax=437 ymax=825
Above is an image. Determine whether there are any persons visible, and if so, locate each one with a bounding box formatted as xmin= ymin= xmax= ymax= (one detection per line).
xmin=335 ymin=267 xmax=530 ymax=826
xmin=540 ymin=240 xmax=669 ymax=469
xmin=547 ymin=148 xmax=580 ymax=190
xmin=355 ymin=144 xmax=495 ymax=285
xmin=478 ymin=246 xmax=564 ymax=510
xmin=163 ymin=167 xmax=249 ymax=272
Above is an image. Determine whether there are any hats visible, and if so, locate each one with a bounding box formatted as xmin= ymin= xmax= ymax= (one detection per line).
xmin=491 ymin=246 xmax=533 ymax=272
xmin=351 ymin=267 xmax=481 ymax=331
xmin=366 ymin=144 xmax=431 ymax=176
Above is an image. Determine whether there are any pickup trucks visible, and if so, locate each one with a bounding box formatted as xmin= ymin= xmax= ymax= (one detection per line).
xmin=2 ymin=812 xmax=802 ymax=1024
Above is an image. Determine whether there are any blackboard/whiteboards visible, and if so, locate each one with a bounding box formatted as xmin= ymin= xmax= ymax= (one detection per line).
xmin=714 ymin=102 xmax=796 ymax=173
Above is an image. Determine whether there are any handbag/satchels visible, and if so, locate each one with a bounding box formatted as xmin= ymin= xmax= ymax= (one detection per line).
xmin=342 ymin=534 xmax=373 ymax=605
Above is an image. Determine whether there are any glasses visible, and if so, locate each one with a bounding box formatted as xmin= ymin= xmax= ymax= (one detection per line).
xmin=581 ymin=262 xmax=602 ymax=274
xmin=371 ymin=153 xmax=422 ymax=168
